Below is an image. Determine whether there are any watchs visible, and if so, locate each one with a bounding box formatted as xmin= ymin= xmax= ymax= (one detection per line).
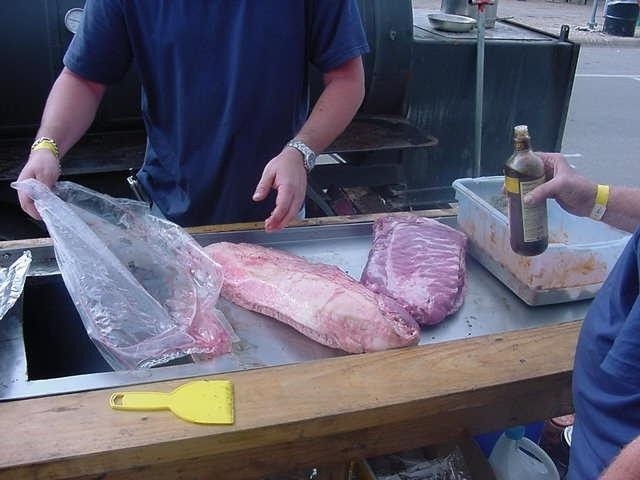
xmin=285 ymin=138 xmax=317 ymax=177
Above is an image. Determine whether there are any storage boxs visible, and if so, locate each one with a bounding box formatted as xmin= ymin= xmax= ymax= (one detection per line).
xmin=341 ymin=429 xmax=499 ymax=479
xmin=449 ymin=171 xmax=634 ymax=309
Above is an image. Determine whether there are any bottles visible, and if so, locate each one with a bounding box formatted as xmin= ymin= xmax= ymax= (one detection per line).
xmin=506 ymin=126 xmax=552 ymax=257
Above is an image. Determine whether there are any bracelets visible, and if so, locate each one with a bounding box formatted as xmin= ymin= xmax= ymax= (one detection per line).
xmin=587 ymin=181 xmax=612 ymax=221
xmin=30 ymin=135 xmax=61 ymax=163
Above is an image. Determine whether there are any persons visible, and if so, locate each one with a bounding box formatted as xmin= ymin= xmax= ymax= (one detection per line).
xmin=500 ymin=149 xmax=640 ymax=480
xmin=16 ymin=0 xmax=373 ymax=235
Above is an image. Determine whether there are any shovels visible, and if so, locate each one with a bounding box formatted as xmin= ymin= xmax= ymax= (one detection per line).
xmin=109 ymin=380 xmax=234 ymax=424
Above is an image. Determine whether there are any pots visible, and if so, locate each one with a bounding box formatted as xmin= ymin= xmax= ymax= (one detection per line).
xmin=427 ymin=12 xmax=515 ymax=33
